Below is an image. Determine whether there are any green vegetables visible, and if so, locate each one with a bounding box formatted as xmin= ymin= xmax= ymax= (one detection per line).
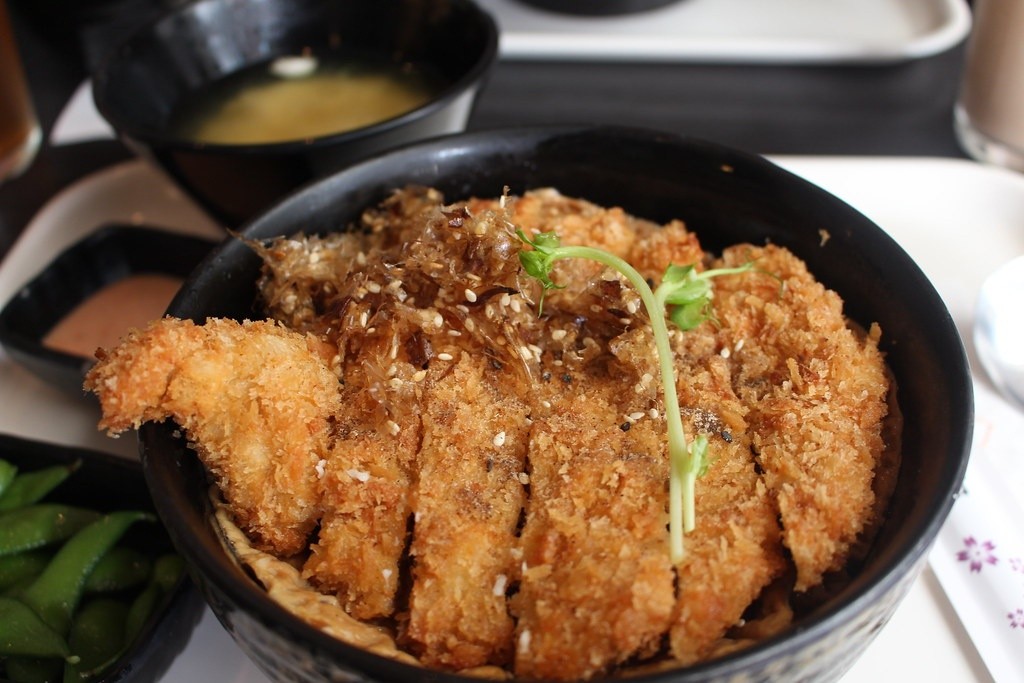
xmin=514 ymin=227 xmax=787 ymax=565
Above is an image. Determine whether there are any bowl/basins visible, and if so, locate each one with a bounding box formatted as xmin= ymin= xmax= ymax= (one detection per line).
xmin=146 ymin=121 xmax=976 ymax=683
xmin=90 ymin=0 xmax=500 ymax=242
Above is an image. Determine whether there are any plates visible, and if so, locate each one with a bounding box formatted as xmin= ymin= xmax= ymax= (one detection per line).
xmin=0 ymin=220 xmax=222 ymax=394
xmin=1 ymin=428 xmax=203 ymax=681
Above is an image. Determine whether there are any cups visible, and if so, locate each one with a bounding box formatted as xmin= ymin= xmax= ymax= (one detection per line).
xmin=954 ymin=0 xmax=1024 ymax=173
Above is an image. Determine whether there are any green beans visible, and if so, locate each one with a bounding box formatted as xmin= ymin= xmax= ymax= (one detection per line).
xmin=0 ymin=455 xmax=185 ymax=683
